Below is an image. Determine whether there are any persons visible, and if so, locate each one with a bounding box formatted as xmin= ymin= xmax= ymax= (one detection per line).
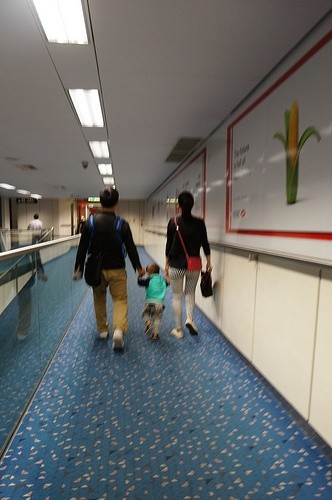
xmin=73 ymin=187 xmax=144 ymax=353
xmin=78 ymin=215 xmax=87 ymax=234
xmin=27 ymin=214 xmax=44 ymax=245
xmin=163 ymin=191 xmax=212 ymax=339
xmin=139 ymin=264 xmax=170 ymax=342
xmin=10 ymin=244 xmax=49 ymax=342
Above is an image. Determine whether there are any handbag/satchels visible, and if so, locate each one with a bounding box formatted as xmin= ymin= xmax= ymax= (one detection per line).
xmin=199 ymin=270 xmax=214 ymax=298
xmin=186 ymin=256 xmax=203 ymax=274
xmin=84 ymin=258 xmax=103 ymax=288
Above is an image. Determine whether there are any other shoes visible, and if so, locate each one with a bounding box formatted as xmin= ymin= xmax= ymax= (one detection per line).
xmin=143 ymin=323 xmax=152 ymax=336
xmin=150 ymin=333 xmax=160 ymax=342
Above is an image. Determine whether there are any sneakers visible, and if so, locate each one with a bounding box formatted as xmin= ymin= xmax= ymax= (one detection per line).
xmin=99 ymin=330 xmax=110 ymax=339
xmin=170 ymin=329 xmax=185 ymax=340
xmin=112 ymin=328 xmax=125 ymax=351
xmin=185 ymin=318 xmax=198 ymax=336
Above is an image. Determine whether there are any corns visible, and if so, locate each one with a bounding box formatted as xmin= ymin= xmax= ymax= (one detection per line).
xmin=272 ymin=101 xmax=318 ymax=204
